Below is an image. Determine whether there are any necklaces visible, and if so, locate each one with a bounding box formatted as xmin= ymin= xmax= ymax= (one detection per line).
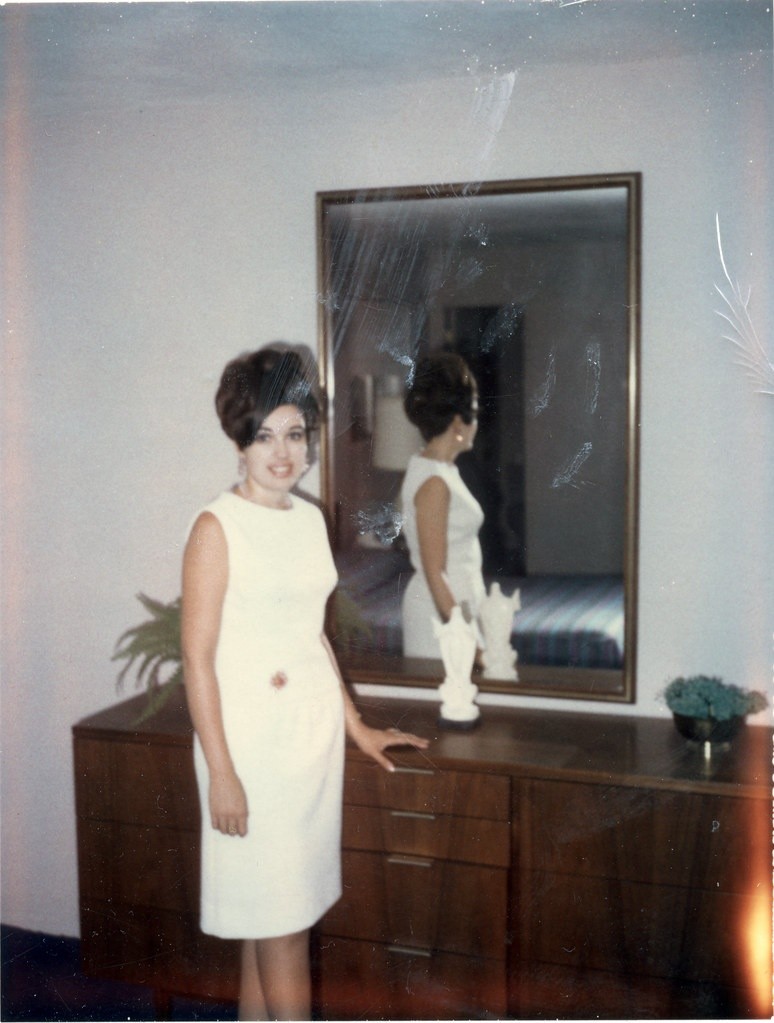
xmin=237 ymin=483 xmax=249 ymax=498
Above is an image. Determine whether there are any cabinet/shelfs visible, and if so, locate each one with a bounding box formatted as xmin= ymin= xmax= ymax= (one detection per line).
xmin=75 ymin=683 xmax=773 ymax=1020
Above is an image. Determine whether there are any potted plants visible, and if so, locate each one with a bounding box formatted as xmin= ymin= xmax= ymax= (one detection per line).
xmin=662 ymin=675 xmax=753 ymax=742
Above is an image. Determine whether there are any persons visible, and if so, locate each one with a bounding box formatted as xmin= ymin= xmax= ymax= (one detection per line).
xmin=397 ymin=354 xmax=488 ymax=669
xmin=178 ymin=342 xmax=427 ymax=1021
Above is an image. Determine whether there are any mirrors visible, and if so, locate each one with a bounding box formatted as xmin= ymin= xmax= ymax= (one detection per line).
xmin=309 ymin=173 xmax=640 ymax=704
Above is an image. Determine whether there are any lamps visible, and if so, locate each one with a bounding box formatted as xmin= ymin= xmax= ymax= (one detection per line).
xmin=374 ymin=396 xmax=421 ymax=471
